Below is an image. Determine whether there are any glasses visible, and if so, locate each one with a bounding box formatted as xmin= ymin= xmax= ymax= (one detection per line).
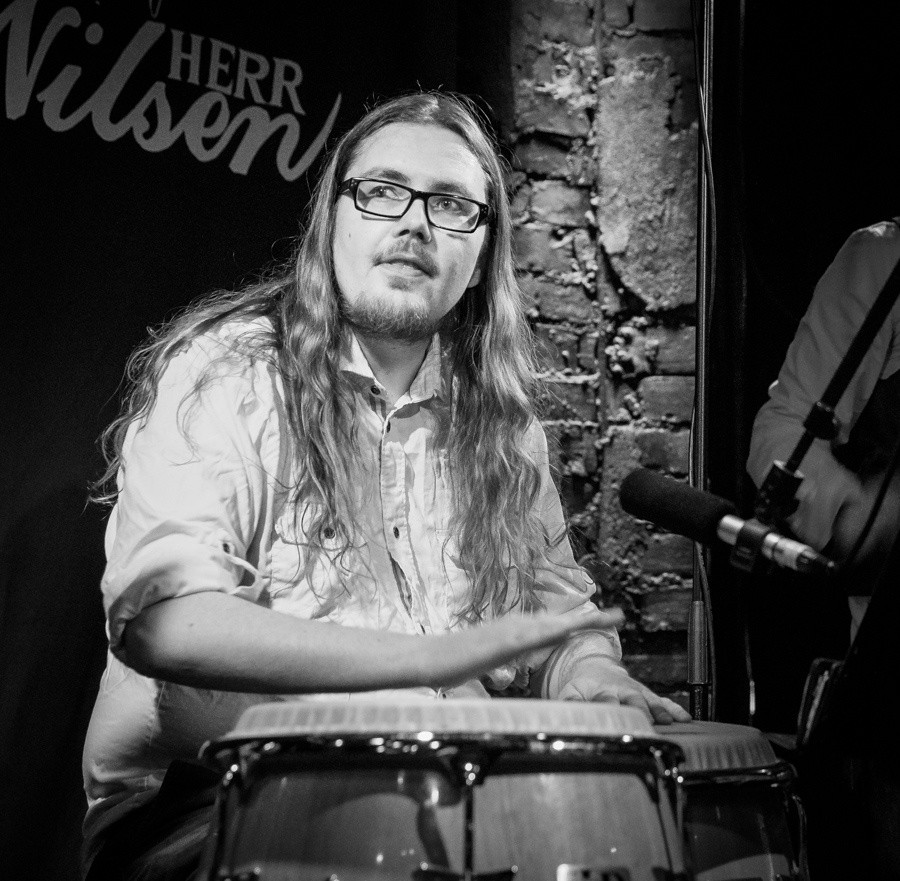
xmin=338 ymin=177 xmax=493 ymax=233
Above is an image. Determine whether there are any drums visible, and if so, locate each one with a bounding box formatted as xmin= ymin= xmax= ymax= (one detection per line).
xmin=198 ymin=692 xmax=687 ymax=880
xmin=654 ymin=719 xmax=814 ymax=881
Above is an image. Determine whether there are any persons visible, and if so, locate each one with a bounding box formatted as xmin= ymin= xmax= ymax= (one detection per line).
xmin=79 ymin=92 xmax=692 ymax=881
xmin=747 ymin=215 xmax=900 ymax=878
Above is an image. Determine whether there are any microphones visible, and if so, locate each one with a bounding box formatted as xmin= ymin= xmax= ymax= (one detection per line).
xmin=618 ymin=467 xmax=834 ymax=575
